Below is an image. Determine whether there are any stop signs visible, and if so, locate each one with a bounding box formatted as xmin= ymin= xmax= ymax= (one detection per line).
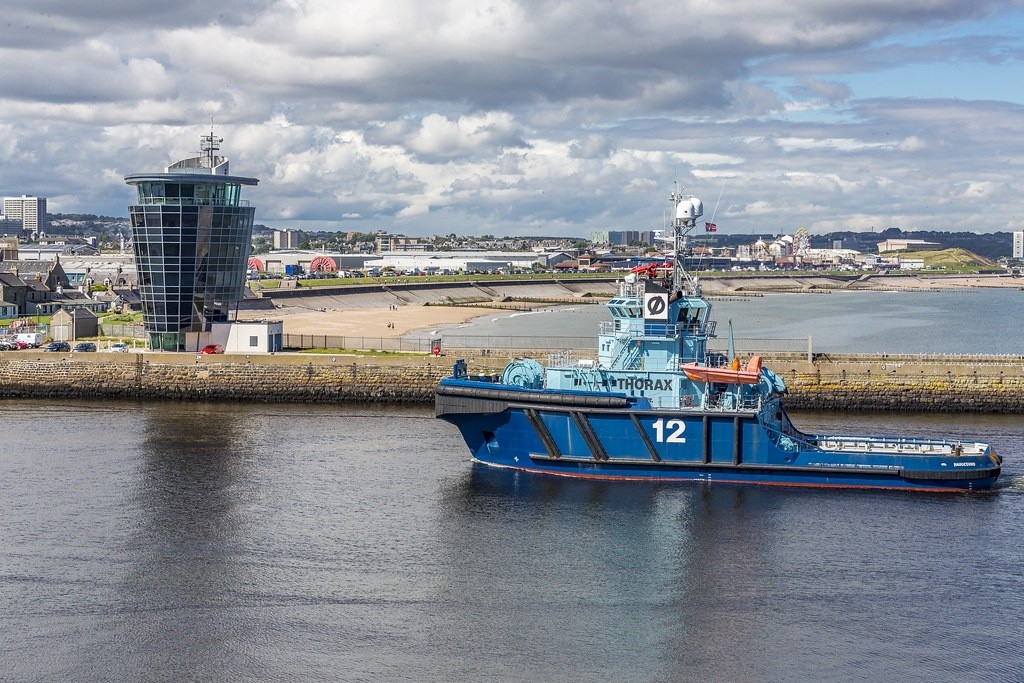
xmin=433 ymin=346 xmax=440 ymax=356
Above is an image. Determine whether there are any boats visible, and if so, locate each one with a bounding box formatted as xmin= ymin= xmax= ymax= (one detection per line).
xmin=430 ymin=175 xmax=1002 ymax=495
xmin=674 ymin=355 xmax=765 ymax=383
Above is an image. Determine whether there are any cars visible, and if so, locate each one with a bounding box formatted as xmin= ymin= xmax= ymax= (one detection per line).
xmin=72 ymin=342 xmax=97 ymax=353
xmin=247 ymin=266 xmax=612 ymax=278
xmin=703 ymin=263 xmax=849 ymax=272
xmin=199 ymin=344 xmax=224 ymax=354
xmin=0 ymin=338 xmax=33 ymax=352
xmin=109 ymin=343 xmax=129 ymax=354
xmin=43 ymin=342 xmax=71 ymax=353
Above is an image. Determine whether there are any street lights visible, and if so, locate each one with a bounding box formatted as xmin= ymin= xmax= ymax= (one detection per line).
xmin=69 ymin=309 xmax=77 ymax=349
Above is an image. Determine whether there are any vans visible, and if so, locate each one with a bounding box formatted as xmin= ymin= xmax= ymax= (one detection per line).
xmin=10 ymin=333 xmax=44 ymax=348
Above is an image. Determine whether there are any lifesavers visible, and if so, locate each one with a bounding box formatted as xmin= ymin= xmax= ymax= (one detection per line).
xmin=684 ymin=396 xmax=692 ymax=406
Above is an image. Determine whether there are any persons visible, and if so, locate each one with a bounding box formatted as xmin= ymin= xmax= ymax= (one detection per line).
xmin=387 ymin=321 xmax=394 ymax=329
xmin=390 ymin=304 xmax=398 ymax=312
